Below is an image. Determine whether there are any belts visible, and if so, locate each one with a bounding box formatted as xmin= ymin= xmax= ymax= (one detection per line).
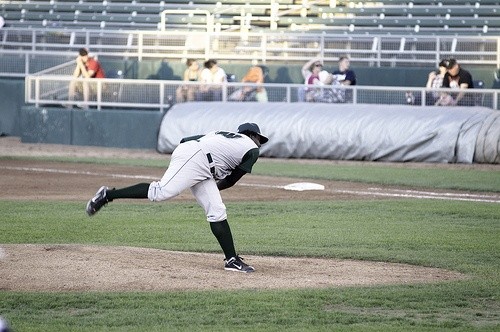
xmin=206 ymin=153 xmax=215 ymax=174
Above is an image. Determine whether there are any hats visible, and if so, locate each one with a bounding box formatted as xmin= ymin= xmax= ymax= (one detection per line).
xmin=237 ymin=123 xmax=269 ymax=144
xmin=439 ymin=59 xmax=449 ymax=68
xmin=446 ymin=59 xmax=457 ymax=70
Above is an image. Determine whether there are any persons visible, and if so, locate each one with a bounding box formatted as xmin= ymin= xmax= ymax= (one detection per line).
xmin=426 ymin=59 xmax=477 ymax=107
xmin=175 ymin=58 xmax=228 ymax=104
xmin=86 ymin=122 xmax=269 ymax=273
xmin=301 ymin=56 xmax=356 ymax=103
xmin=490 ymin=64 xmax=500 ymax=110
xmin=66 ymin=48 xmax=107 ymax=110
xmin=233 ymin=66 xmax=268 ymax=102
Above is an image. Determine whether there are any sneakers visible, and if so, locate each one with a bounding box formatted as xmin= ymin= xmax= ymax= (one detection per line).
xmin=405 ymin=90 xmax=415 ymax=105
xmin=223 ymin=253 xmax=256 ymax=273
xmin=86 ymin=186 xmax=114 ymax=216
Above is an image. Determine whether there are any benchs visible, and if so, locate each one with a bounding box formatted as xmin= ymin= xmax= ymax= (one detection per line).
xmin=0 ymin=0 xmax=500 ymax=57
